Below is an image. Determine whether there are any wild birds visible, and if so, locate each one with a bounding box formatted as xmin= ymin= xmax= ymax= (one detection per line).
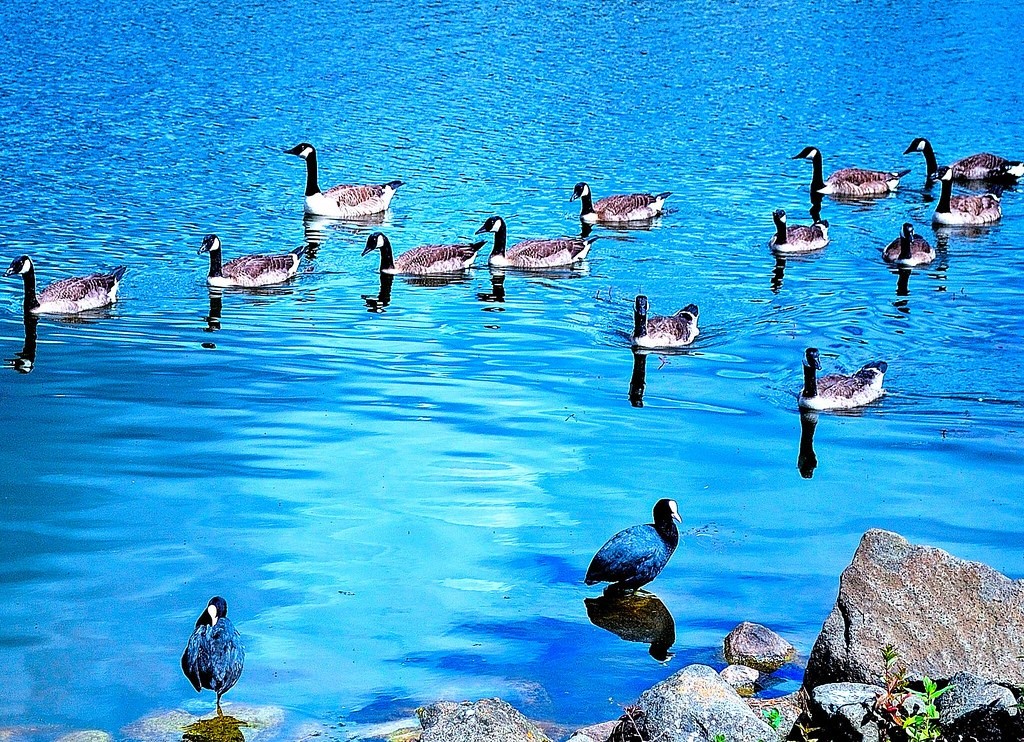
xmin=628 ymin=343 xmax=692 ymax=408
xmin=358 ymin=265 xmax=478 ymax=313
xmin=583 ymin=585 xmax=677 ymax=663
xmin=477 ymin=256 xmax=590 ymax=313
xmin=583 ymin=498 xmax=683 ymax=593
xmin=795 ymin=395 xmax=884 ymax=479
xmin=2 ymin=304 xmax=115 ymax=373
xmin=4 ymin=254 xmax=128 ymax=313
xmin=196 ymin=234 xmax=306 ymax=287
xmin=361 ymin=232 xmax=489 ymax=275
xmin=200 ymin=273 xmax=297 ymax=349
xmin=769 ymin=137 xmax=1024 ymax=265
xmin=629 ymin=294 xmax=700 ymax=349
xmin=796 ymin=348 xmax=888 ymax=410
xmin=284 ymin=142 xmax=407 ymax=215
xmin=296 ymin=210 xmax=386 ymax=274
xmin=180 ymin=597 xmax=245 ymax=703
xmin=474 ymin=216 xmax=599 ymax=268
xmin=569 ymin=181 xmax=673 ymax=222
